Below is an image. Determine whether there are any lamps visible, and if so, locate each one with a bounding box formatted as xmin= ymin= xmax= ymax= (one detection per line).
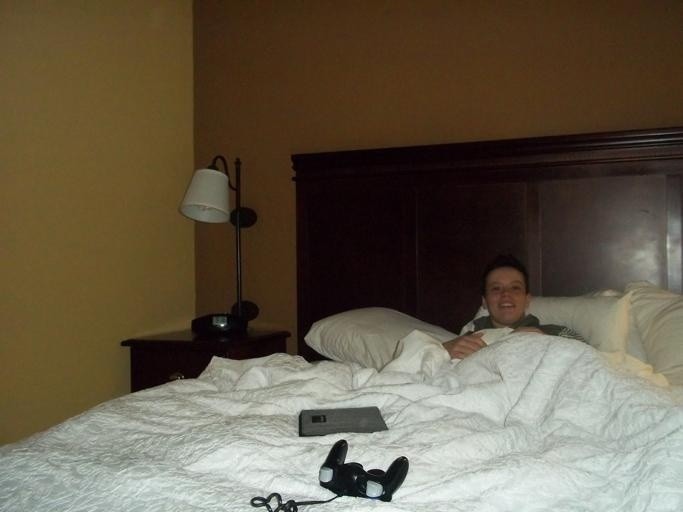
xmin=178 ymin=155 xmax=260 ymax=335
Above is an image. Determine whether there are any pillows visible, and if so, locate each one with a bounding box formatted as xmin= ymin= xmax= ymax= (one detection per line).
xmin=459 ymin=290 xmax=635 ymax=354
xmin=303 ymin=306 xmax=459 ymax=372
xmin=623 ymin=278 xmax=683 ymax=373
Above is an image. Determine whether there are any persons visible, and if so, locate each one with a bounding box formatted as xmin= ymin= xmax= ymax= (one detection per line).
xmin=441 ymin=259 xmax=591 ymax=359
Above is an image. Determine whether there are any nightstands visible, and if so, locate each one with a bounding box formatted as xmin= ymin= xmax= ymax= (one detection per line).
xmin=120 ymin=328 xmax=291 ymax=394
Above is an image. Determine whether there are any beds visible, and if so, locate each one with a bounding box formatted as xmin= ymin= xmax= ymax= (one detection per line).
xmin=0 ymin=127 xmax=682 ymax=511
